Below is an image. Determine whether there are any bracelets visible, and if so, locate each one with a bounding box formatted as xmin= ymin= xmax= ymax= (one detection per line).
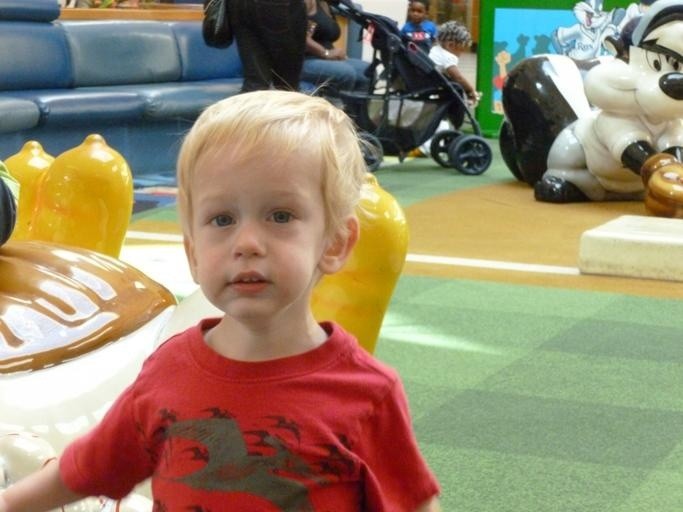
xmin=322 ymin=47 xmax=330 ymax=59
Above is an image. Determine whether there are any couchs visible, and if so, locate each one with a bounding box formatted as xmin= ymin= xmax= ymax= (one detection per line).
xmin=1 ymin=1 xmax=323 ymax=179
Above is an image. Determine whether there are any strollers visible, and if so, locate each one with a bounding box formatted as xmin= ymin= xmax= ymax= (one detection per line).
xmin=330 ymin=0 xmax=492 ymax=176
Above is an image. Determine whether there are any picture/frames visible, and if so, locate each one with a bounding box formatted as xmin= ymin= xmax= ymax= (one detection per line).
xmin=475 ymin=2 xmax=642 ymax=140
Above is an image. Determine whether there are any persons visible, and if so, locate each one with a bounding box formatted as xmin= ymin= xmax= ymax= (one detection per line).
xmin=428 ymin=21 xmax=479 ymax=107
xmin=303 ymin=1 xmax=380 ymax=134
xmin=222 ymin=1 xmax=307 ymax=92
xmin=399 ymin=1 xmax=437 ymax=56
xmin=0 ymin=89 xmax=443 ymax=512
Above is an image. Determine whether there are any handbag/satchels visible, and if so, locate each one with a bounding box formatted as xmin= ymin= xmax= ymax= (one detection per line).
xmin=201 ymin=1 xmax=234 ymax=49
